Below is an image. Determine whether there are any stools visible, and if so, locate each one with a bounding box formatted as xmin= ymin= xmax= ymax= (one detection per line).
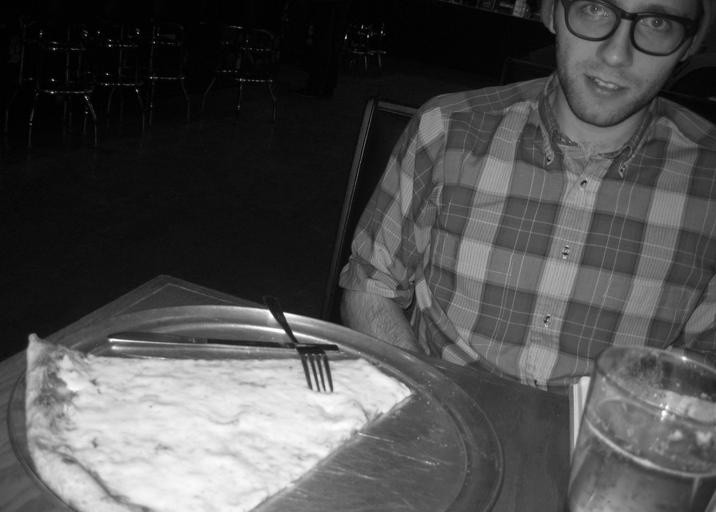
xmin=18 ymin=0 xmax=388 ymax=156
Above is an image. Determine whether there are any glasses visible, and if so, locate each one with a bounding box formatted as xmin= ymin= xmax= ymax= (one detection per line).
xmin=559 ymin=0 xmax=700 ymax=58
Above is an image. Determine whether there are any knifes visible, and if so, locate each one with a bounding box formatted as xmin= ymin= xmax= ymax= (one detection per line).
xmin=104 ymin=332 xmax=340 ymax=353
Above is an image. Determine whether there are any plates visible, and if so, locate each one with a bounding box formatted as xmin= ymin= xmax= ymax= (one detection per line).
xmin=6 ymin=302 xmax=505 ymax=512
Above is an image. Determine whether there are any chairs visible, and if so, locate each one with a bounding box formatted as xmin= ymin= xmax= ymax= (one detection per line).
xmin=322 ymin=97 xmax=419 ymax=325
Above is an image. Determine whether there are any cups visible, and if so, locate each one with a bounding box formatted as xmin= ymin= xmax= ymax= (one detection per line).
xmin=561 ymin=347 xmax=716 ymax=512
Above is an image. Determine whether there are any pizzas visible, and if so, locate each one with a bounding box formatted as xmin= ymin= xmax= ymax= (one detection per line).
xmin=20 ymin=328 xmax=415 ymax=512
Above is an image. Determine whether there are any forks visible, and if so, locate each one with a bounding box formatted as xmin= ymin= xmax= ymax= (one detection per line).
xmin=264 ymin=294 xmax=334 ymax=395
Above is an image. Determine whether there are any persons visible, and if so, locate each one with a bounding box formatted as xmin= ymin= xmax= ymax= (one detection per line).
xmin=332 ymin=2 xmax=716 ymax=408
